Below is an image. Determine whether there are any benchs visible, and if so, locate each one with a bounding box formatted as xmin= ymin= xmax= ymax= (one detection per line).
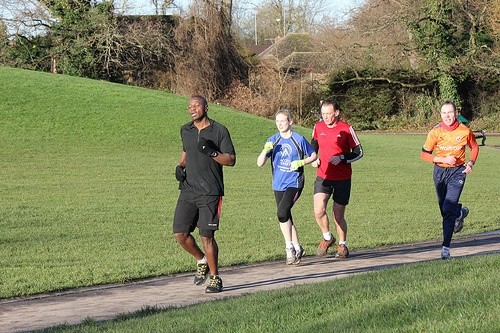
xmin=473 ymin=130 xmax=486 ymax=145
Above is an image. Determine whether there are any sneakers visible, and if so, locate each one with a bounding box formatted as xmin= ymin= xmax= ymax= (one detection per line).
xmin=335 ymin=244 xmax=350 ymax=258
xmin=441 ymin=246 xmax=450 ymax=260
xmin=292 ymin=245 xmax=306 ymax=265
xmin=194 ymin=263 xmax=210 ymax=286
xmin=285 ymin=246 xmax=296 ymax=265
xmin=205 ymin=275 xmax=223 ymax=293
xmin=453 ymin=207 xmax=469 ymax=233
xmin=317 ymin=234 xmax=336 ymax=257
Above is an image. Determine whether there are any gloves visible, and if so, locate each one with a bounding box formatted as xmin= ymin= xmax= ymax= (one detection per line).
xmin=197 ymin=139 xmax=214 ymax=158
xmin=176 ymin=165 xmax=186 ymax=183
xmin=290 ymin=160 xmax=304 ymax=170
xmin=263 ymin=142 xmax=274 ymax=154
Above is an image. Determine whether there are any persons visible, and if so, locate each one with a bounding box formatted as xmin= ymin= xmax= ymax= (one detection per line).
xmin=310 ymin=100 xmax=364 ymax=258
xmin=257 ymin=110 xmax=317 ymax=265
xmin=173 ymin=96 xmax=236 ymax=294
xmin=421 ymin=101 xmax=480 ymax=259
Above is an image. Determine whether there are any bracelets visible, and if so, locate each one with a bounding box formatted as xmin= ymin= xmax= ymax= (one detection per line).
xmin=469 ymin=160 xmax=475 ymax=166
